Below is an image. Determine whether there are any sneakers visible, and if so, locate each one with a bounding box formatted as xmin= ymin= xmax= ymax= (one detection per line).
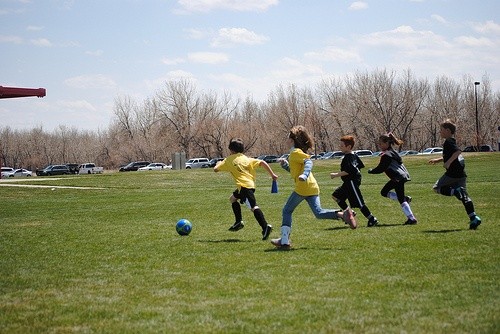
xmin=344 ymin=209 xmax=357 ymax=224
xmin=343 ymin=208 xmax=357 ymax=229
xmin=229 ymin=220 xmax=244 ymax=231
xmin=270 ymin=238 xmax=291 ymax=250
xmin=366 ymin=216 xmax=378 ymax=227
xmin=468 ymin=215 xmax=481 ymax=230
xmin=454 ymin=186 xmax=468 ymax=205
xmin=262 ymin=224 xmax=272 ymax=240
xmin=402 ymin=218 xmax=417 ymax=225
xmin=404 ymin=195 xmax=412 ymax=203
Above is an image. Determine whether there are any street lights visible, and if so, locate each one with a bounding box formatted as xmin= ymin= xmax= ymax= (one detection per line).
xmin=474 ymin=81 xmax=480 ymax=146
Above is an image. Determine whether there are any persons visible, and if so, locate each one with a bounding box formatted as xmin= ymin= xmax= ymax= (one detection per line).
xmin=425 ymin=121 xmax=482 ymax=231
xmin=331 ymin=135 xmax=378 ymax=227
xmin=215 ymin=137 xmax=277 ymax=240
xmin=368 ymin=133 xmax=418 ymax=226
xmin=270 ymin=126 xmax=357 ymax=249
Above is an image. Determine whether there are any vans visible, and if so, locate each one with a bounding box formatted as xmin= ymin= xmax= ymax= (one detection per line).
xmin=185 ymin=157 xmax=224 ymax=169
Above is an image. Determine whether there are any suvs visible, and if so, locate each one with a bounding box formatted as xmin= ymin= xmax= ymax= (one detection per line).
xmin=36 ymin=162 xmax=104 ymax=176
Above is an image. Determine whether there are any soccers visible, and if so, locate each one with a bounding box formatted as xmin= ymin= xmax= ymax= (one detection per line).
xmin=175 ymin=219 xmax=192 ymax=236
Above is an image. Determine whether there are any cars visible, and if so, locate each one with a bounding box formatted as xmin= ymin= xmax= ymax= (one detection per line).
xmin=355 ymin=149 xmax=381 ymax=157
xmin=0 ymin=167 xmax=32 ymax=178
xmin=309 ymin=150 xmax=343 ymax=161
xmin=459 ymin=145 xmax=494 ymax=152
xmin=119 ymin=161 xmax=173 ymax=172
xmin=257 ymin=154 xmax=289 ymax=163
xmin=400 ymin=147 xmax=443 ymax=156
